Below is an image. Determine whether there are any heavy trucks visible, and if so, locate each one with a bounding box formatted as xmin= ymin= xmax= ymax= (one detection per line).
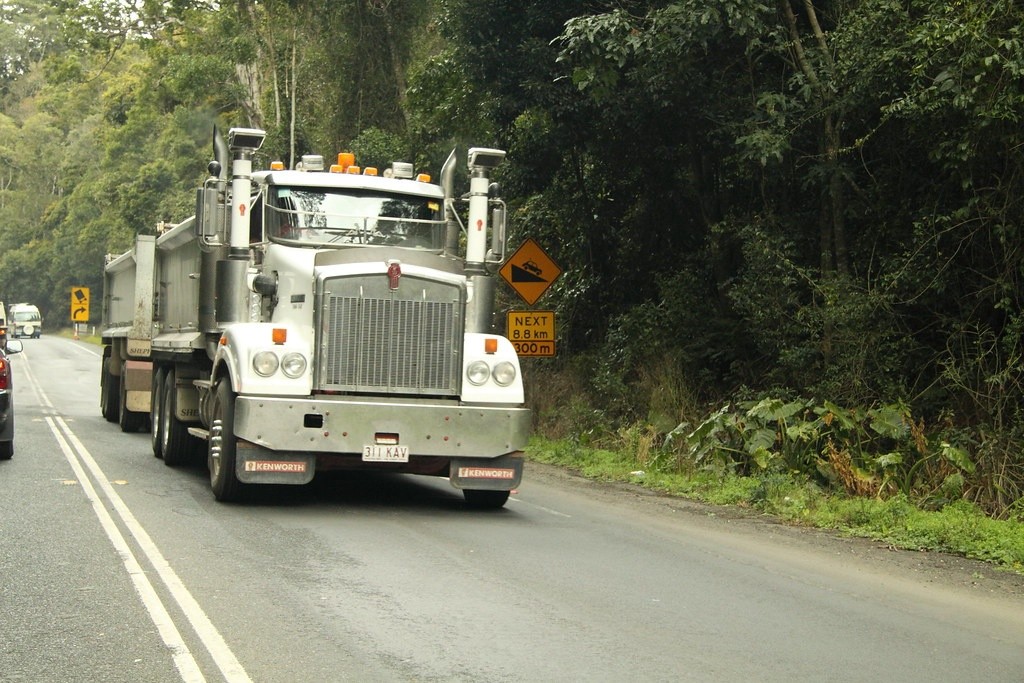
xmin=96 ymin=122 xmax=530 ymax=508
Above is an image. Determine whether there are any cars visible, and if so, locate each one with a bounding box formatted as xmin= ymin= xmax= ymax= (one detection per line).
xmin=0 ymin=339 xmax=24 ymax=460
xmin=0 ymin=301 xmax=9 ymax=350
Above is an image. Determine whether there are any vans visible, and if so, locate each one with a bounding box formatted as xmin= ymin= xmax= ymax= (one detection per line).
xmin=6 ymin=302 xmax=43 ymax=339
